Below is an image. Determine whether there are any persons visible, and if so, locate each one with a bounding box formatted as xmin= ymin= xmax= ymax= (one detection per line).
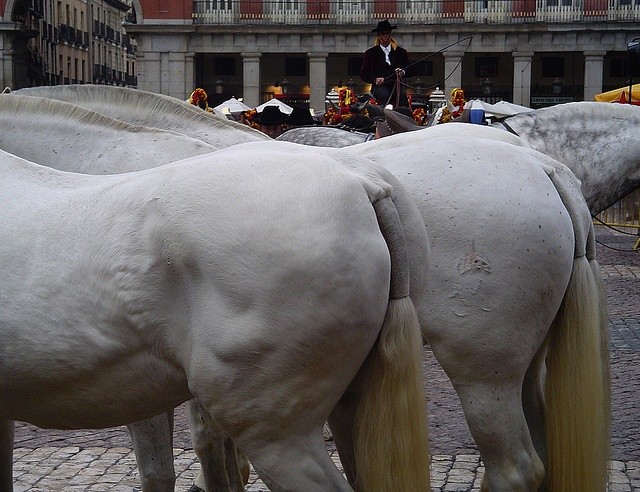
xmin=360 ymin=21 xmax=409 ymax=108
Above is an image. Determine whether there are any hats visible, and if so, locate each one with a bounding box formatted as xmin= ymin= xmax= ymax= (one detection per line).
xmin=372 ymin=21 xmax=396 ymax=32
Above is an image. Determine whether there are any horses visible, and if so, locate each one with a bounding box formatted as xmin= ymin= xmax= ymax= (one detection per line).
xmin=276 ymin=102 xmax=640 ymax=441
xmin=0 ymin=141 xmax=432 ymax=492
